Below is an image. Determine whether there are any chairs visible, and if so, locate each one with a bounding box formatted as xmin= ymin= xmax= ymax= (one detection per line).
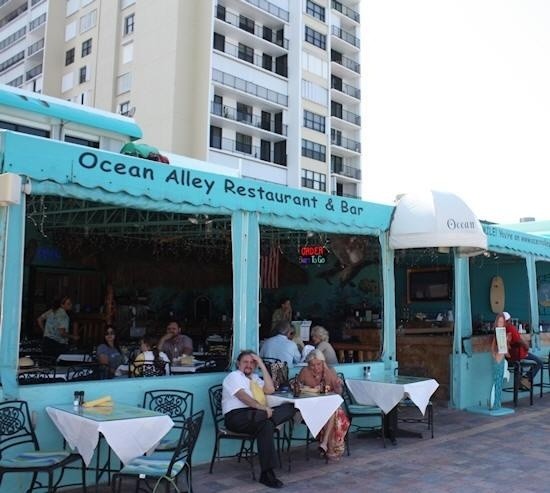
xmin=11 ymin=333 xmax=204 ymax=383
xmin=109 ymin=411 xmax=204 ymax=493
xmin=2 ymin=398 xmax=94 ymax=493
xmin=205 ymin=342 xmax=440 ymax=482
xmin=110 ymin=388 xmax=195 ymax=491
xmin=501 ymin=352 xmax=550 ymax=411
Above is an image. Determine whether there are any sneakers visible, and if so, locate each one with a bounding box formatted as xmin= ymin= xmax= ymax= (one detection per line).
xmin=520 ymin=377 xmax=532 ymax=389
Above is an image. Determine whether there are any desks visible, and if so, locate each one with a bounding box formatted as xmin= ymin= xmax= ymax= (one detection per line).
xmin=40 ymin=401 xmax=174 ymax=493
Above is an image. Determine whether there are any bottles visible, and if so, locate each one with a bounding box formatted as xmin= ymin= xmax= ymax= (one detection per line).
xmin=173 ymin=347 xmax=179 ymax=362
xmin=363 ymin=366 xmax=371 ymax=378
xmin=397 ymin=305 xmax=412 ymax=329
xmin=73 ymin=391 xmax=84 ymax=406
xmin=293 ymin=378 xmax=300 ymax=398
xmin=320 ymin=377 xmax=326 ymax=394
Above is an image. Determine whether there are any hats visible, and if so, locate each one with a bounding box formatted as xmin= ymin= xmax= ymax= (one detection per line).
xmin=503 ymin=312 xmax=511 ymax=321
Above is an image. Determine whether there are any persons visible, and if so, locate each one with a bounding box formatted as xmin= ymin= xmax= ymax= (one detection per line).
xmin=298 ymin=349 xmax=350 ymax=460
xmin=38 ymin=295 xmax=80 ymax=366
xmin=504 ymin=312 xmax=543 ymax=391
xmin=491 ymin=314 xmax=512 ymax=410
xmin=98 ymin=297 xmax=338 ymax=377
xmin=222 ymin=351 xmax=296 ymax=488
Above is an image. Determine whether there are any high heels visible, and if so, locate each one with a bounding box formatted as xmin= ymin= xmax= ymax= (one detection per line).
xmin=319 ymin=443 xmax=328 ymax=456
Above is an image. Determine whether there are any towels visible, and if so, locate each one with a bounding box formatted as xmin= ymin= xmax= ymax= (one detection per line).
xmin=82 ymin=394 xmax=114 ymax=407
xmin=250 ymin=378 xmax=266 ymax=405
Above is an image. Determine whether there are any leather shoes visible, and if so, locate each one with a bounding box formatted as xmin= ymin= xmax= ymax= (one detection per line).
xmin=259 ymin=472 xmax=284 ymax=488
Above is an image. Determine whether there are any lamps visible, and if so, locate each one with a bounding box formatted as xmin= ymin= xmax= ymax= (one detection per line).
xmin=186 ymin=211 xmax=214 ymax=224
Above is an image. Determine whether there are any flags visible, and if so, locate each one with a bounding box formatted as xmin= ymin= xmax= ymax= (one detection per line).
xmin=260 ymin=239 xmax=279 ymax=289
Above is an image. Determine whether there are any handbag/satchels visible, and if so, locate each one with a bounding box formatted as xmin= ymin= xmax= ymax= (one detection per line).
xmin=261 ymin=358 xmax=289 ymax=390
